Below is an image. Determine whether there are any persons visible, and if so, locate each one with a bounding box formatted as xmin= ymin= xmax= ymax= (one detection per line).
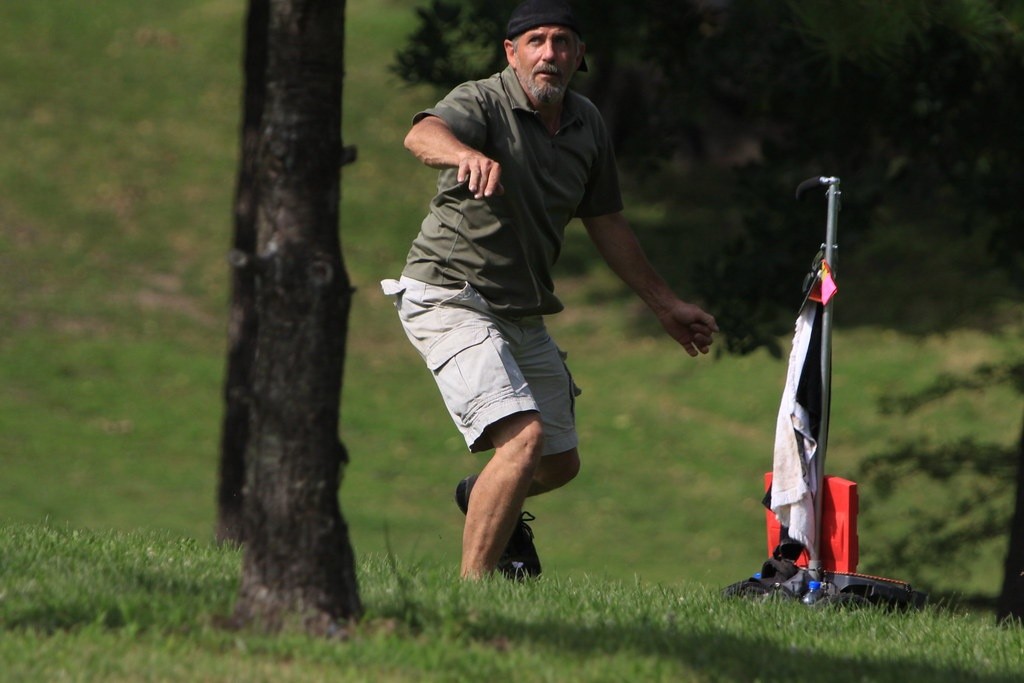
xmin=380 ymin=0 xmax=720 ymax=586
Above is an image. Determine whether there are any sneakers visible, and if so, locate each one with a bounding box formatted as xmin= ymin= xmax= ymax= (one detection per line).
xmin=456 ymin=474 xmax=542 ymax=585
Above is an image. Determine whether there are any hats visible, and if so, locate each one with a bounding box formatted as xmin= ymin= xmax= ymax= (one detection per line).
xmin=506 ymin=0 xmax=589 ymax=72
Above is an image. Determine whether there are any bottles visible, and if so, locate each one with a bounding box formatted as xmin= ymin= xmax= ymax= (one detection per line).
xmin=802 ymin=581 xmax=822 ymax=604
